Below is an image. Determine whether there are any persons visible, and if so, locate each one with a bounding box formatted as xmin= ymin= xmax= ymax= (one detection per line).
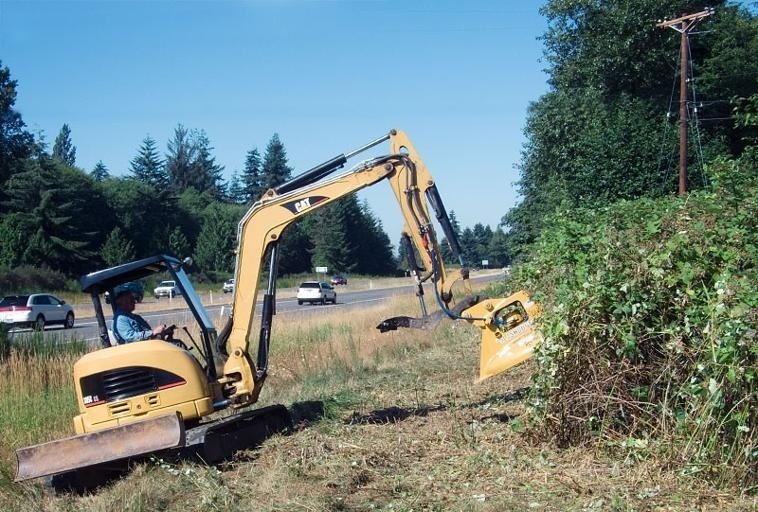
xmin=110 ymin=289 xmax=168 ymax=345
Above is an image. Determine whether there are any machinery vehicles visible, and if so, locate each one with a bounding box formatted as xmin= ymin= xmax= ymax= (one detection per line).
xmin=15 ymin=129 xmax=545 ymax=496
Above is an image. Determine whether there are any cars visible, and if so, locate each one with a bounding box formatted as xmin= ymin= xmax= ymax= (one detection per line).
xmin=154 ymin=281 xmax=181 ymax=298
xmin=297 ymin=281 xmax=337 ymax=305
xmin=0 ymin=293 xmax=74 ymax=331
xmin=332 ymin=277 xmax=347 ymax=285
xmin=223 ymin=279 xmax=235 ymax=293
